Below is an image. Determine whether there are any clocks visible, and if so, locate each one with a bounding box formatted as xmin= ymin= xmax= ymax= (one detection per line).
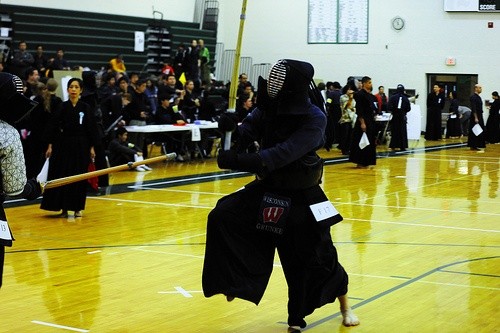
xmin=392 ymin=18 xmax=405 ymax=30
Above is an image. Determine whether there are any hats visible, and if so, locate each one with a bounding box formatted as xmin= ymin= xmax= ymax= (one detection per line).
xmin=159 ymin=93 xmax=173 ymax=100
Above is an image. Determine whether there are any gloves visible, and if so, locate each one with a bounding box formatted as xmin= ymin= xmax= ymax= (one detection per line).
xmin=217 ymin=110 xmax=239 ymax=137
xmin=217 ymin=149 xmax=264 ymax=176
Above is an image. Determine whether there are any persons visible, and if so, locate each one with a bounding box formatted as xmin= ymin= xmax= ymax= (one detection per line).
xmin=40 ymin=78 xmax=95 ymax=218
xmin=0 ymin=39 xmax=256 ymax=172
xmin=0 ymin=73 xmax=39 ymax=288
xmin=202 ymin=60 xmax=360 ymax=333
xmin=424 ymin=83 xmax=472 ymax=140
xmin=467 ymin=84 xmax=486 ymax=151
xmin=485 ymin=91 xmax=500 ymax=144
xmin=316 ymin=76 xmax=421 ymax=169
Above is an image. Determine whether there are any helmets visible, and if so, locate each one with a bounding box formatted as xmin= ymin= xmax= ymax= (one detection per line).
xmin=268 ymin=60 xmax=314 ymax=105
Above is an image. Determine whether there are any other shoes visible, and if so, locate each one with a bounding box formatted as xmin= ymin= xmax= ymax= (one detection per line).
xmin=135 ymin=165 xmax=152 ymax=172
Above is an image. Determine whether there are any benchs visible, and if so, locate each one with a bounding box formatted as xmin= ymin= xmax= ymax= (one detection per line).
xmin=0 ymin=3 xmax=229 ymax=115
